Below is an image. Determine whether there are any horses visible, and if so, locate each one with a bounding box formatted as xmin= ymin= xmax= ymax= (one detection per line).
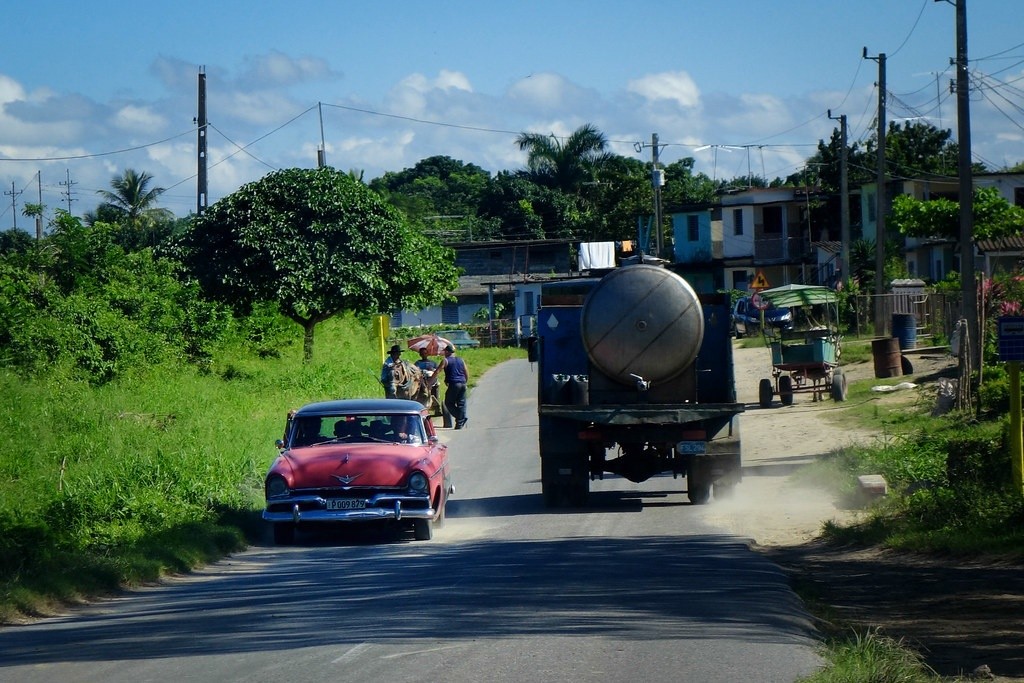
xmin=384 ymin=360 xmax=434 ymax=410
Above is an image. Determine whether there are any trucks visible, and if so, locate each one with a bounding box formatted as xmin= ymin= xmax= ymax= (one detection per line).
xmin=536 ymin=262 xmax=746 ymax=509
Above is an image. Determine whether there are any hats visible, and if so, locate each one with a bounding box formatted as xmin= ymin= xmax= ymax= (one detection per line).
xmin=387 ymin=345 xmax=405 ymax=354
xmin=360 ymin=419 xmax=393 ymax=434
xmin=442 ymin=344 xmax=453 ymax=351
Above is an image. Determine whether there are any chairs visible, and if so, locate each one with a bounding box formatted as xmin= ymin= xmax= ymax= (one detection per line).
xmin=406 ymin=418 xmax=422 ymax=444
xmin=368 ymin=420 xmax=386 ymax=439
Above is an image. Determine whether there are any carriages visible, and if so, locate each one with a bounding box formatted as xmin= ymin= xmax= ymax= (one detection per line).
xmin=758 ymin=283 xmax=848 ymax=408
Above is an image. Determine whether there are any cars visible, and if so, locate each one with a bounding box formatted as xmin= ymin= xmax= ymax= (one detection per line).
xmin=264 ymin=399 xmax=457 ymax=545
xmin=435 ymin=330 xmax=480 ymax=350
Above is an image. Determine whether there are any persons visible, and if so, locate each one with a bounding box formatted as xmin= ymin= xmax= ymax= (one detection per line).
xmin=296 ymin=409 xmax=327 ymax=447
xmin=431 ymin=345 xmax=469 ymax=429
xmin=333 ymin=418 xmax=388 ymax=441
xmin=395 ymin=383 xmax=410 ymax=402
xmin=381 ymin=345 xmax=410 ymax=400
xmin=414 ymin=347 xmax=442 ymax=417
xmin=389 ymin=414 xmax=420 ymax=444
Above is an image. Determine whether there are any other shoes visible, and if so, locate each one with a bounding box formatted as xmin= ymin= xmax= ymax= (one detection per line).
xmin=455 ymin=417 xmax=468 ymax=429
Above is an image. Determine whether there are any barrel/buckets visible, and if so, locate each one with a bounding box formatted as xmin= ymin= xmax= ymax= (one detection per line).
xmin=892 ymin=313 xmax=916 ymax=349
xmin=872 ymin=338 xmax=902 ymax=378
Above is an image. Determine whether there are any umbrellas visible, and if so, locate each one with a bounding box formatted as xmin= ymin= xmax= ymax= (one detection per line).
xmin=408 ymin=335 xmax=457 ymax=357
